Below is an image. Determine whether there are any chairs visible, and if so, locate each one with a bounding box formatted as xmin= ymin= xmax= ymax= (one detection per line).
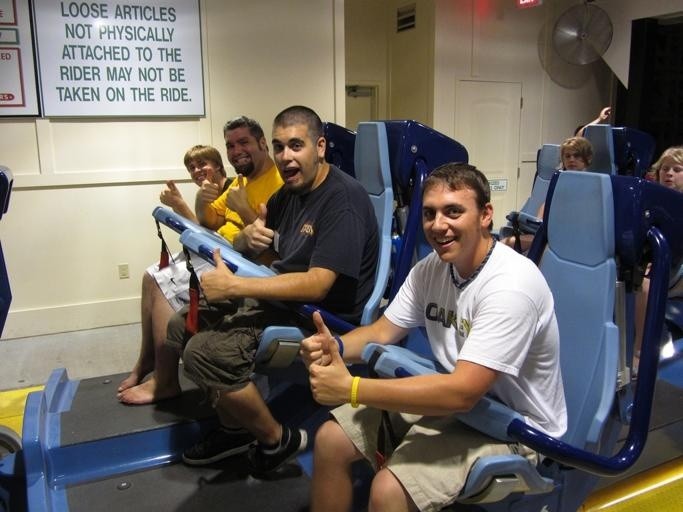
xmin=153 ymin=119 xmax=682 ymax=512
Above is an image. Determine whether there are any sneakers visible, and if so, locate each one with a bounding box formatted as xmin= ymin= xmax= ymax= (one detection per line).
xmin=245 ymin=423 xmax=308 ymax=481
xmin=181 ymin=424 xmax=259 ymax=466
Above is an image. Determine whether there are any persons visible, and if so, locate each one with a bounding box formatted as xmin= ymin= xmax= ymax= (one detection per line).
xmin=298 ymin=165 xmax=569 ymax=509
xmin=162 ymin=105 xmax=381 ymax=474
xmin=558 ymin=139 xmax=590 ymax=172
xmin=629 ymin=146 xmax=683 ymax=379
xmin=163 ymin=141 xmax=232 ymax=224
xmin=497 ymin=104 xmax=614 ymax=252
xmin=117 ymin=113 xmax=278 ymax=406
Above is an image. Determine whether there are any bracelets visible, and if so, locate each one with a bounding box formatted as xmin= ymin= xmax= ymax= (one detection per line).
xmin=333 ymin=333 xmax=345 ymax=363
xmin=347 ymin=376 xmax=362 ymax=409
